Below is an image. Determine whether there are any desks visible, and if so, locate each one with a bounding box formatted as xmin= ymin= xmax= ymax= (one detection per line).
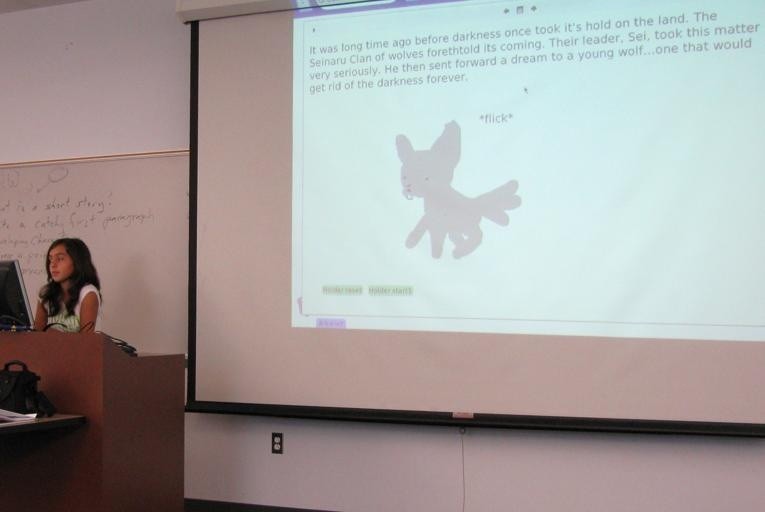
xmin=0 ymin=412 xmax=85 ymax=435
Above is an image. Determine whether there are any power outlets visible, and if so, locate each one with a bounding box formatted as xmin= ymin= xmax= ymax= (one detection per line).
xmin=272 ymin=433 xmax=282 ymax=453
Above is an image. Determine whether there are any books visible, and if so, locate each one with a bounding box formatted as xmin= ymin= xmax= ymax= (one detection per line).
xmin=1 ymin=409 xmax=37 ymax=422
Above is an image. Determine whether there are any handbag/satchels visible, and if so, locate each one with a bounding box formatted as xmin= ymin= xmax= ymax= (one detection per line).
xmin=0 ymin=360 xmax=41 ymax=415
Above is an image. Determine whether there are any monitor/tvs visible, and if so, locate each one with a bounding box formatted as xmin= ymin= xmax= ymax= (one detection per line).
xmin=0 ymin=259 xmax=35 ymax=326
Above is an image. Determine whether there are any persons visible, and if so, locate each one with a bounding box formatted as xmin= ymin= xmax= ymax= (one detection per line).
xmin=32 ymin=236 xmax=103 ymax=334
